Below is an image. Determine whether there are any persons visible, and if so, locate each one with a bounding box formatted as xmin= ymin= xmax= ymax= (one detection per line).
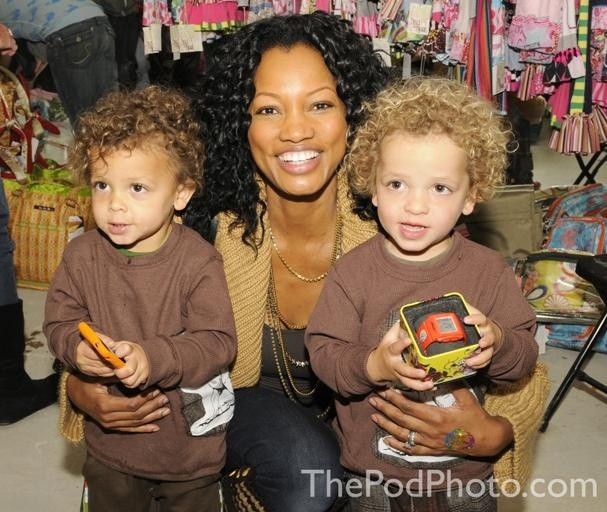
xmin=0 ymin=0 xmax=120 ymax=133
xmin=0 ymin=24 xmax=18 ymax=57
xmin=0 ymin=179 xmax=59 ymax=427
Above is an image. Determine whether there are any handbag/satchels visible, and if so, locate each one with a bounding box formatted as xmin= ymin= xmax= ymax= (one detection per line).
xmin=0 ymin=69 xmax=93 ymax=292
xmin=466 ymin=184 xmax=607 ymax=352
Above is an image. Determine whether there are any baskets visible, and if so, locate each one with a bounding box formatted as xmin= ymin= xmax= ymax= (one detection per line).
xmin=548 ymin=104 xmax=607 ymax=156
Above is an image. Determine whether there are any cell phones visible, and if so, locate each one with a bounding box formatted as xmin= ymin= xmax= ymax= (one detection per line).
xmin=77 ymin=321 xmax=125 ymax=369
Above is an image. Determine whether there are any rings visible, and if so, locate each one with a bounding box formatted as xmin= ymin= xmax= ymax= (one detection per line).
xmin=7 ymin=28 xmax=15 ymax=36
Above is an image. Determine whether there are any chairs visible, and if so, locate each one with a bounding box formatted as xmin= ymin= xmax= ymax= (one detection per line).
xmin=512 ymin=139 xmax=605 ymax=431
